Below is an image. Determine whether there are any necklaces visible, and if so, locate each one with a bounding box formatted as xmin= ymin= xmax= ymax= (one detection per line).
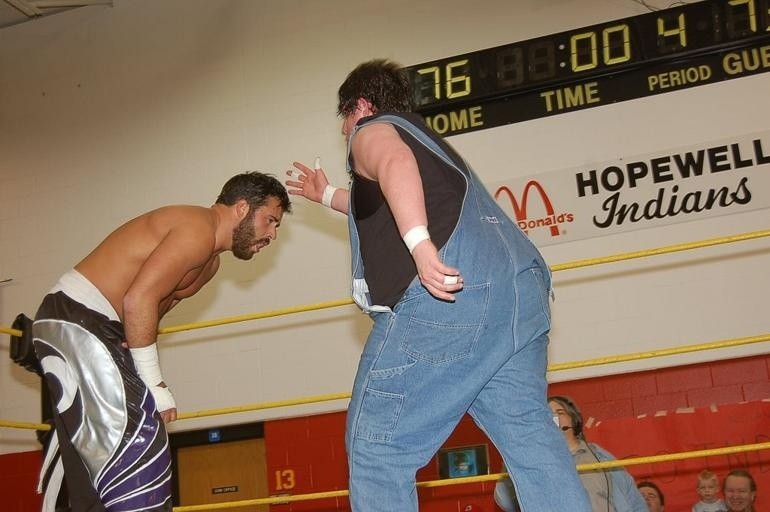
xmin=443 ymin=276 xmax=457 ymax=285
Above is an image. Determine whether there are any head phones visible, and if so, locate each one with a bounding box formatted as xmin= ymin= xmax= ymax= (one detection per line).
xmin=548 ymin=397 xmax=583 ymax=436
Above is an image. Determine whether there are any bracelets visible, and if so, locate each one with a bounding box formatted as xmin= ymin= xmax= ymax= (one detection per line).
xmin=402 ymin=225 xmax=431 ymax=253
xmin=321 ymin=184 xmax=342 ymax=208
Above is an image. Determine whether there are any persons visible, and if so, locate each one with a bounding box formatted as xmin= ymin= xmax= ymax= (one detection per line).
xmin=723 ymin=470 xmax=759 ymax=511
xmin=692 ymin=469 xmax=728 ymax=511
xmin=31 ymin=170 xmax=293 ymax=512
xmin=285 ymin=60 xmax=592 ymax=511
xmin=637 ymin=481 xmax=665 ymax=511
xmin=494 ymin=393 xmax=649 ymax=511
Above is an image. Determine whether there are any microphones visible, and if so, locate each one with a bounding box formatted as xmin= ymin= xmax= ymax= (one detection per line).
xmin=561 ymin=425 xmax=574 ymax=431
